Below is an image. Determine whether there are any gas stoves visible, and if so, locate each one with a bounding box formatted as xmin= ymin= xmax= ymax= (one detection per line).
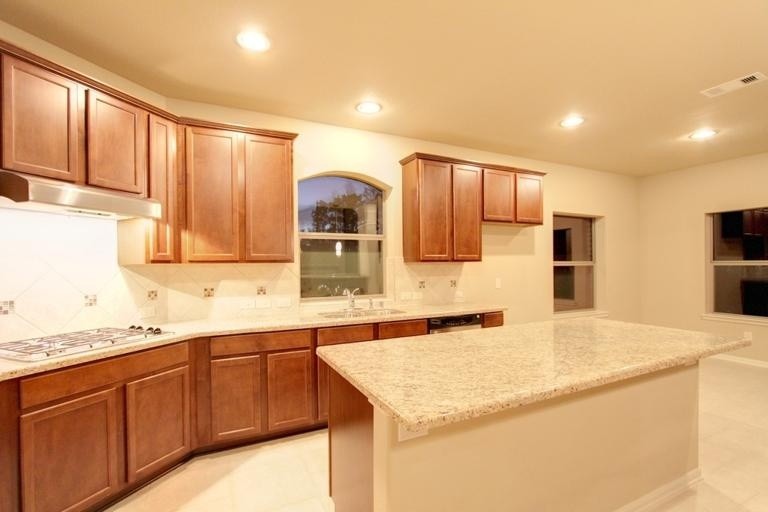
xmin=0 ymin=325 xmax=167 ymax=363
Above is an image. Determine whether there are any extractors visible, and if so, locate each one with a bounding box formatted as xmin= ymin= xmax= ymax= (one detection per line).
xmin=0 ymin=168 xmax=162 ymax=225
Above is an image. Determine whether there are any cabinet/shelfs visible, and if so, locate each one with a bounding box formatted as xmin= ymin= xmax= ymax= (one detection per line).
xmin=182 ymin=106 xmax=300 ymax=265
xmin=0 ymin=52 xmax=151 ymax=201
xmin=162 ymin=304 xmax=510 ymax=456
xmin=3 ymin=325 xmax=194 ymax=511
xmin=397 ymin=152 xmax=484 ymax=262
xmin=482 ymin=163 xmax=548 ymax=224
xmin=115 ymin=106 xmax=180 ymax=266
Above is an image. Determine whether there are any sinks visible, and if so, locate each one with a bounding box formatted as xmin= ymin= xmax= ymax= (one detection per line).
xmin=317 ymin=307 xmax=406 ymax=320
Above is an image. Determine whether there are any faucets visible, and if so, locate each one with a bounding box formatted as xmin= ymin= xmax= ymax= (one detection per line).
xmin=343 ymin=288 xmax=356 ymax=308
xmin=317 ymin=284 xmax=334 ymax=297
